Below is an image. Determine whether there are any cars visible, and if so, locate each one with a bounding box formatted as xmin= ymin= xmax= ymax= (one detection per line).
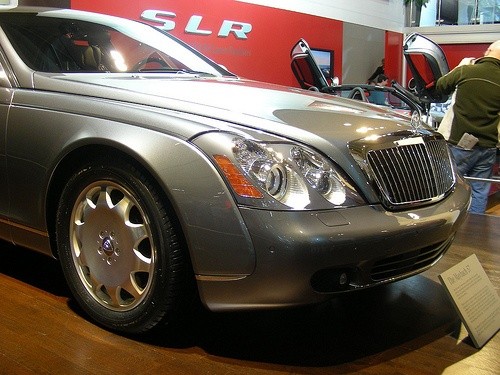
xmin=0 ymin=5 xmax=474 ymax=336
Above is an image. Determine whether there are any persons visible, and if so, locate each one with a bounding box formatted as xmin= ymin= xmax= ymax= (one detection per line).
xmin=365 ymin=81 xmax=376 ymax=97
xmin=370 ymin=75 xmax=391 ymax=107
xmin=436 ymin=40 xmax=500 ymax=215
